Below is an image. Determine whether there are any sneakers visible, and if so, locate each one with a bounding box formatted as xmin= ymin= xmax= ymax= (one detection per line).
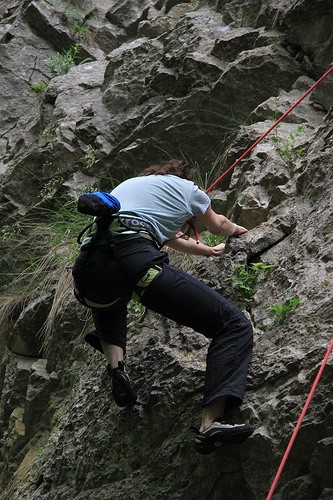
xmin=105 ymin=360 xmax=138 ymax=408
xmin=194 ymin=418 xmax=254 ymax=455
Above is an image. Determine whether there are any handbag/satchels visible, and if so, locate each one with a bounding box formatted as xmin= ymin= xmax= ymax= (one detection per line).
xmin=78 ymin=192 xmax=121 ymax=216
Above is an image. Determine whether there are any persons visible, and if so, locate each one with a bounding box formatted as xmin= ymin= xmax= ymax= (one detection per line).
xmin=72 ymin=165 xmax=257 ymax=454
